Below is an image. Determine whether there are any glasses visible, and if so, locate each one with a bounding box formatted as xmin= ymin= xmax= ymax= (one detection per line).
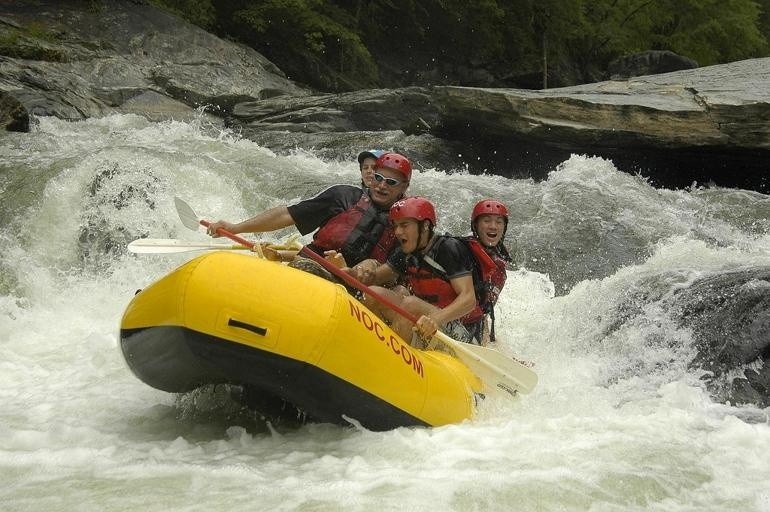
xmin=374 ymin=172 xmax=400 ymax=186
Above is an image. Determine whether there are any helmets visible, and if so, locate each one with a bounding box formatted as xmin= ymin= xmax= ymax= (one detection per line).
xmin=390 ymin=196 xmax=436 ymax=225
xmin=472 ymin=199 xmax=508 ymax=222
xmin=376 ymin=152 xmax=411 ymax=181
xmin=358 ymin=150 xmax=384 ymax=159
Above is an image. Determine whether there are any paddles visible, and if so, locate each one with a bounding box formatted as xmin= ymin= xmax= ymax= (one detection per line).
xmin=286 ymin=234 xmax=538 ymax=402
xmin=127 ymin=238 xmax=298 ymax=253
xmin=174 ymin=196 xmax=254 ymax=250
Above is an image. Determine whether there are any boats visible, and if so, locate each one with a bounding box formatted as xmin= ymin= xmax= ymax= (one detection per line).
xmin=121 ymin=252 xmax=484 ymax=432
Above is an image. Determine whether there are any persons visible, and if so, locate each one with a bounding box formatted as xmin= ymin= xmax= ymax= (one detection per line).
xmin=458 ymin=200 xmax=511 ymax=343
xmin=324 ymin=197 xmax=476 ymax=351
xmin=358 ymin=149 xmax=386 ymax=189
xmin=206 ymin=153 xmax=413 ymax=281
